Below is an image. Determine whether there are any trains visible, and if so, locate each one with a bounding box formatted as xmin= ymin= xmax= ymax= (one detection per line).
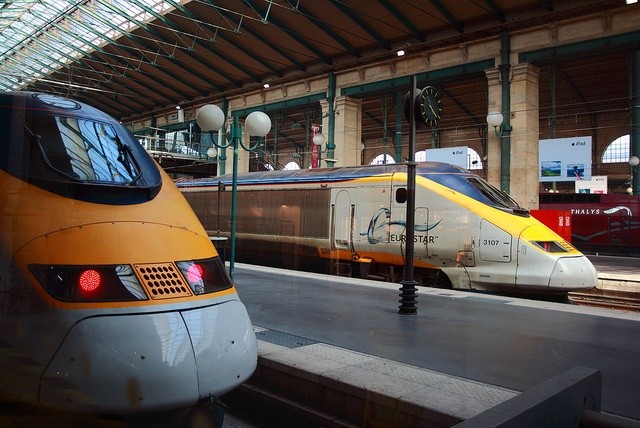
xmin=171 ymin=162 xmax=599 ymax=303
xmin=0 ymin=91 xmax=260 ymax=428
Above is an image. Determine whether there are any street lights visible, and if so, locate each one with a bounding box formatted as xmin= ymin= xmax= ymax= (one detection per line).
xmin=195 ymin=100 xmax=272 ymax=282
